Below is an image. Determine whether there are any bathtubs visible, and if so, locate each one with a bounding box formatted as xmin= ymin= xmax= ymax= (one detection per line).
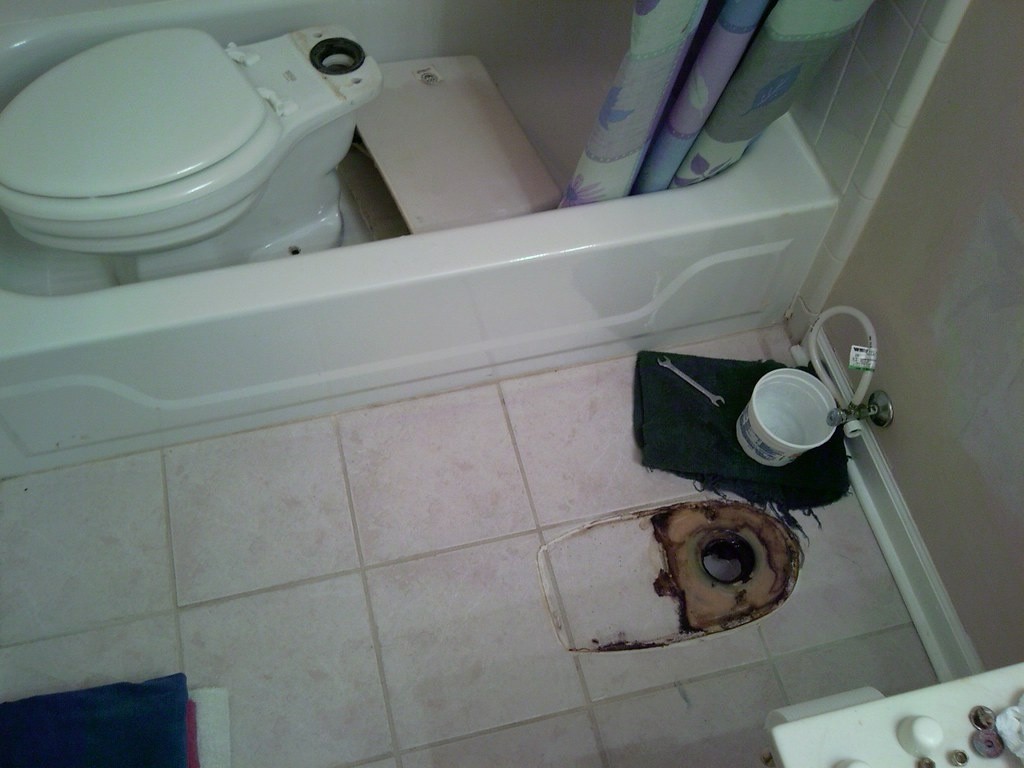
xmin=0 ymin=2 xmax=841 ymax=483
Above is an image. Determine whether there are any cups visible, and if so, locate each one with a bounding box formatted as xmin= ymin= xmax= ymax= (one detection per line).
xmin=737 ymin=368 xmax=837 ymax=467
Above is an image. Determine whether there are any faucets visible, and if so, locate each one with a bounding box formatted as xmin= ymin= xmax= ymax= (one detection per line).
xmin=827 ymin=399 xmax=880 ymax=426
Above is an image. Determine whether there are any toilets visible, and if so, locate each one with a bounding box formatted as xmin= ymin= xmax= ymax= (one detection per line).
xmin=1 ymin=23 xmax=383 ymax=289
